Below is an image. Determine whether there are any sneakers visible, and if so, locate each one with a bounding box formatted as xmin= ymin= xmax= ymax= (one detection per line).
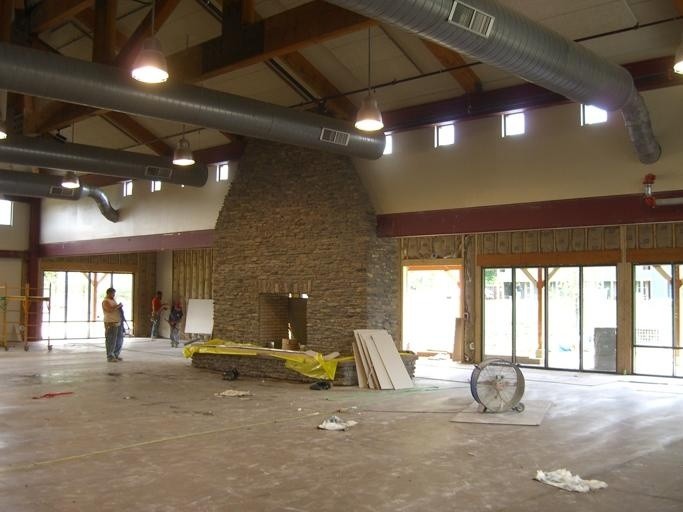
xmin=106 ymin=358 xmax=123 ymax=362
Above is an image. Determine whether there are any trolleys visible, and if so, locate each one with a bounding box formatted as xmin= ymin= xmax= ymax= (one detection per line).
xmin=0 ymin=282 xmax=54 ymax=352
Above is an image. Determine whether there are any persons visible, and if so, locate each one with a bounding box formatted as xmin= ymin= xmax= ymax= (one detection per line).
xmin=102 ymin=288 xmax=124 ymax=362
xmin=169 ymin=301 xmax=183 ymax=348
xmin=151 ymin=290 xmax=169 ymax=339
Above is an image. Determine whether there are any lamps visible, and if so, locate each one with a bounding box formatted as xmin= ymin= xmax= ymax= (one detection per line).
xmin=62 ymin=120 xmax=80 ymax=189
xmin=131 ymin=3 xmax=169 ymax=83
xmin=353 ymin=16 xmax=384 ymax=132
xmin=173 ymin=125 xmax=195 ymax=167
